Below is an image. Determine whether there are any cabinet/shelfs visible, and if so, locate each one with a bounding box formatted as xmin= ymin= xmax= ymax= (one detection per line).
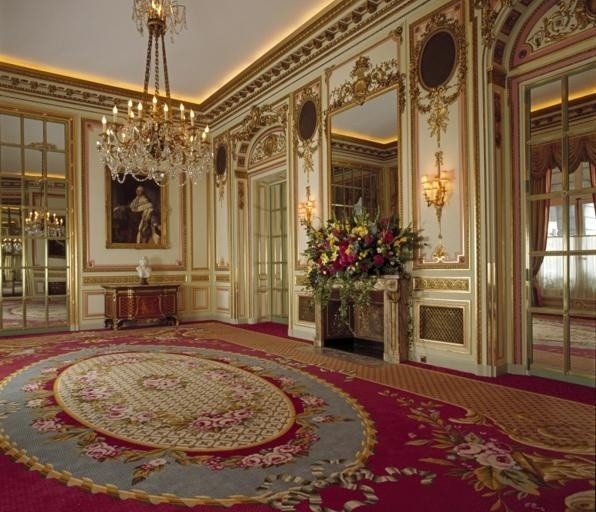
xmin=101 ymin=283 xmax=181 ymax=331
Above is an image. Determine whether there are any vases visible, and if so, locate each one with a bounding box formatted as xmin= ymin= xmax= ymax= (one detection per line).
xmin=136 ymin=256 xmax=153 ymax=285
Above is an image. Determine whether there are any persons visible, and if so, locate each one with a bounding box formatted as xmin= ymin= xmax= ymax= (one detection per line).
xmin=136 ymin=257 xmax=152 ymax=279
xmin=128 ymin=186 xmax=162 ymax=245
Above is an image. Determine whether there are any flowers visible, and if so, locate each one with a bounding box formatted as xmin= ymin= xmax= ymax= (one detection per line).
xmin=298 ymin=199 xmax=431 ymax=336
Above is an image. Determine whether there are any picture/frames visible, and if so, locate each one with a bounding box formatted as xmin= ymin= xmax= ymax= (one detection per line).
xmin=47 ymin=216 xmax=65 ymax=258
xmin=104 ymin=166 xmax=170 ymax=249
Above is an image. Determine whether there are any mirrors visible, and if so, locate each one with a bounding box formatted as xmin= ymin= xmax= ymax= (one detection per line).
xmin=521 ymin=57 xmax=594 ymax=385
xmin=0 ymin=102 xmax=77 ymax=338
xmin=328 ymin=83 xmax=403 ymax=227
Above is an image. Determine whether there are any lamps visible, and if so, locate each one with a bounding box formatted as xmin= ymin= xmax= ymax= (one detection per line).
xmin=298 ymin=198 xmax=316 ymax=223
xmin=421 ymin=171 xmax=450 ymax=223
xmin=96 ymin=1 xmax=216 ymax=185
xmin=2 ymin=208 xmax=24 ymax=252
xmin=22 ymin=178 xmax=66 ymax=239
